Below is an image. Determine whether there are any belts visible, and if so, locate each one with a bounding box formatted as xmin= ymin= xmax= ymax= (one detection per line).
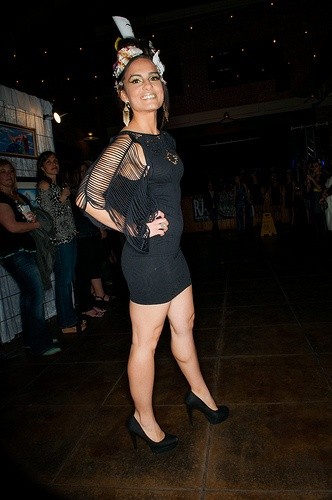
xmin=4 ymin=248 xmax=37 ymax=259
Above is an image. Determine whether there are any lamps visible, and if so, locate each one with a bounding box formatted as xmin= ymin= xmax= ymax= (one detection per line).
xmin=42 ymin=110 xmax=67 ymax=124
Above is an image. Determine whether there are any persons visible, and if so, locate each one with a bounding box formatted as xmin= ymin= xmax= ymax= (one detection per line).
xmin=76 ymin=37 xmax=230 ymax=454
xmin=305 ymin=160 xmax=330 ymax=260
xmin=204 ymin=180 xmax=221 ymax=232
xmin=233 ymin=167 xmax=296 ymax=234
xmin=0 ymin=159 xmax=58 ymax=357
xmin=36 ymin=151 xmax=118 ymax=334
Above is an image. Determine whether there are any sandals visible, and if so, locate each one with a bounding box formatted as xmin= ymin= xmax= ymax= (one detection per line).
xmin=60 ymin=321 xmax=87 ymax=336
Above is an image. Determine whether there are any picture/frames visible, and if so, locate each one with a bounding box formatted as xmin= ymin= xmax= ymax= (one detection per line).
xmin=1 ymin=122 xmax=37 ymax=160
xmin=14 ymin=178 xmax=38 ymax=208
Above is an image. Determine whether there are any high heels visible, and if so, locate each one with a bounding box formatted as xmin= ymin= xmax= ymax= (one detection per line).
xmin=186 ymin=391 xmax=229 ymax=426
xmin=80 ymin=306 xmax=108 ymax=318
xmin=130 ymin=417 xmax=179 ymax=453
xmin=91 ymin=290 xmax=115 ymax=304
